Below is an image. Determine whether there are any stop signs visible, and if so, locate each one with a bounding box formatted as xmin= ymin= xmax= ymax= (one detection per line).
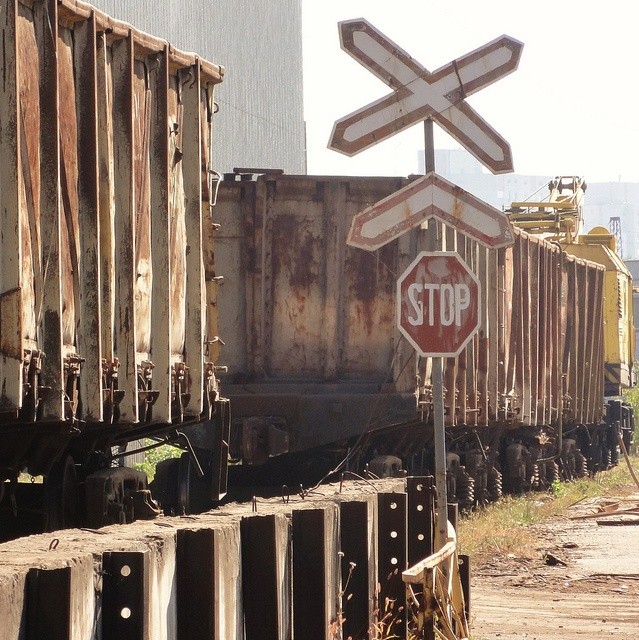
xmin=394 ymin=250 xmax=481 ymax=356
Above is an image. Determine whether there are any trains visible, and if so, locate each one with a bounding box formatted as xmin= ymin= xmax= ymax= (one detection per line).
xmin=0 ymin=0 xmax=632 ymax=640
